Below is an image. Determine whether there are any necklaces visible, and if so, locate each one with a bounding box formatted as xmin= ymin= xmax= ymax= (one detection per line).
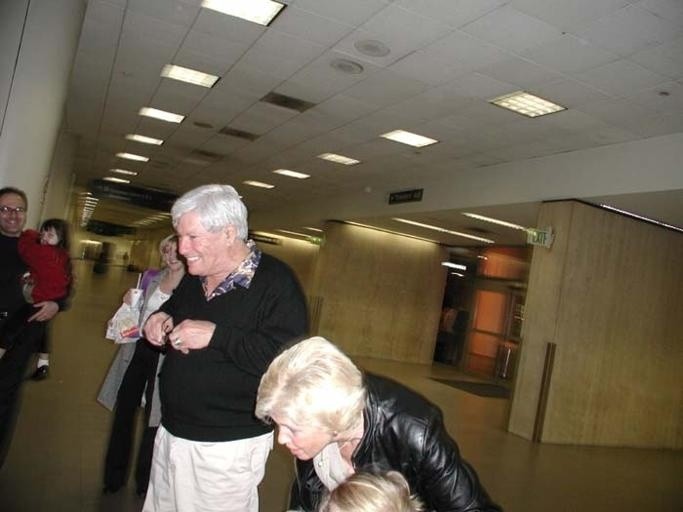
xmin=318 ymin=421 xmax=360 ymax=468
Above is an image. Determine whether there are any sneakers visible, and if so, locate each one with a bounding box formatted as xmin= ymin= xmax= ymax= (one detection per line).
xmin=101 ymin=484 xmax=146 ymax=498
xmin=29 ymin=364 xmax=49 ymax=380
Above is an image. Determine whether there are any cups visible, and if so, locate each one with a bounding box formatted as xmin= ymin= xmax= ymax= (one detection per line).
xmin=128 ymin=288 xmax=143 ymax=310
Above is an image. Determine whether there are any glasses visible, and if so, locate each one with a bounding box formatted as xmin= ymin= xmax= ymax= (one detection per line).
xmin=0 ymin=207 xmax=24 ymax=212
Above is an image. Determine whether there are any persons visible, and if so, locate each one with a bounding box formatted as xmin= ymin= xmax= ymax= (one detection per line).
xmin=432 ymin=302 xmax=472 ymax=364
xmin=97 ymin=233 xmax=185 ymax=502
xmin=252 ymin=334 xmax=504 ymax=512
xmin=14 ymin=216 xmax=71 ymax=382
xmin=323 ymin=466 xmax=425 ymax=512
xmin=134 ymin=183 xmax=312 ymax=512
xmin=0 ymin=186 xmax=71 ymax=475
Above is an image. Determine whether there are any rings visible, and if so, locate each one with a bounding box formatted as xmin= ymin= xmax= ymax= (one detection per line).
xmin=173 ymin=338 xmax=183 ymax=346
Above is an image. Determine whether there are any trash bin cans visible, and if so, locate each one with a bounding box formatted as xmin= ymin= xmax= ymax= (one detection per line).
xmin=493 ymin=343 xmax=518 ymax=380
